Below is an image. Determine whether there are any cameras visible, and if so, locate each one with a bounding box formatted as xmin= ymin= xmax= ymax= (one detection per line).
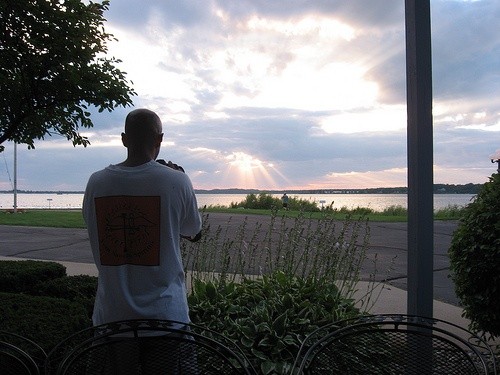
xmin=156 ymin=159 xmax=178 ymax=170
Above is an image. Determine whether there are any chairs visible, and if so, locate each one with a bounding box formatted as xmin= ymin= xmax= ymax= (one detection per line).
xmin=0 ymin=330 xmax=48 ymax=375
xmin=289 ymin=312 xmax=497 ymax=374
xmin=42 ymin=319 xmax=258 ymax=374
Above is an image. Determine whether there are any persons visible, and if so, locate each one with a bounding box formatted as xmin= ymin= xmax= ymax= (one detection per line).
xmin=281 ymin=192 xmax=288 ymax=210
xmin=82 ymin=109 xmax=203 ymax=375
